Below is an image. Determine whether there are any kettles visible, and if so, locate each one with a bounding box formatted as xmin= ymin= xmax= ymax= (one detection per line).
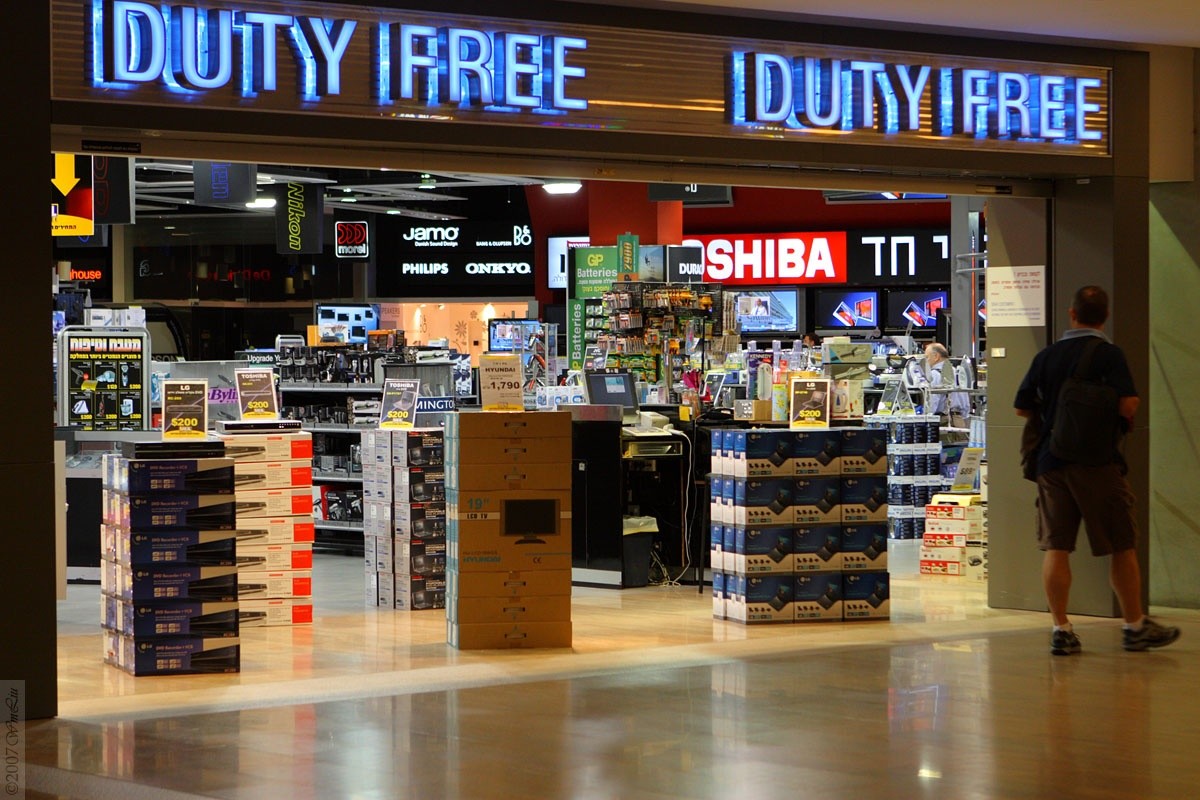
xmin=832 ymin=389 xmax=848 ymax=412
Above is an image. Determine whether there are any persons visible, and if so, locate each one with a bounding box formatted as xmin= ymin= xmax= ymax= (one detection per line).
xmin=751 ymin=298 xmax=768 ymax=316
xmin=803 ymin=334 xmax=816 ymax=347
xmin=924 ymin=342 xmax=970 ymax=443
xmin=1007 ymin=284 xmax=1181 ymax=657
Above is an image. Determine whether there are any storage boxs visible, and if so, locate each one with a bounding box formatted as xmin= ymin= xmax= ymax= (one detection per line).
xmin=98 ymin=409 xmax=987 ymax=677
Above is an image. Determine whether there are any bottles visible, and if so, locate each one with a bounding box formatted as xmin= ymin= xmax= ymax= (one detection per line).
xmin=679 ymin=380 xmax=699 ymax=420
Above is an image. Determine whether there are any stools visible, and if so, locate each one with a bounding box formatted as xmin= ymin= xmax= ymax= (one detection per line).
xmin=931 ymin=493 xmax=981 ymax=507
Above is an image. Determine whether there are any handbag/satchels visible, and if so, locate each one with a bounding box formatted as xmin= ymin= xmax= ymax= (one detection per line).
xmin=1020 ymin=415 xmax=1046 ymax=478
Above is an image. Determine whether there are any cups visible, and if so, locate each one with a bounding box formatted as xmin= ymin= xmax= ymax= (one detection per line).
xmin=58 ymin=261 xmax=71 ymax=281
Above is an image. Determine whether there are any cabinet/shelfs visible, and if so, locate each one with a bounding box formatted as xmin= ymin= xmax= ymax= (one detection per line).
xmin=280 ymin=379 xmax=380 ymax=531
xmin=585 ymin=279 xmax=723 ymax=382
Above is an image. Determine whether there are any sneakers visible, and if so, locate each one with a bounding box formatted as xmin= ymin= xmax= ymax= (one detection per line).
xmin=1120 ymin=618 xmax=1181 ymax=650
xmin=1050 ymin=629 xmax=1081 ymax=654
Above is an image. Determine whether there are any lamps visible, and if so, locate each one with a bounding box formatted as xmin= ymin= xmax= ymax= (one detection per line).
xmin=540 ymin=179 xmax=583 ymax=195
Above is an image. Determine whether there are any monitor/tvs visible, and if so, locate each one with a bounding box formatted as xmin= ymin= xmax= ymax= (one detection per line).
xmin=488 ymin=317 xmax=545 ymax=354
xmin=723 ymin=289 xmax=806 ymax=338
xmin=314 ymin=300 xmax=380 ymax=345
xmin=882 ymin=286 xmax=953 ymax=334
xmin=810 ymin=286 xmax=881 ymax=338
xmin=589 ymin=373 xmax=641 ymax=413
